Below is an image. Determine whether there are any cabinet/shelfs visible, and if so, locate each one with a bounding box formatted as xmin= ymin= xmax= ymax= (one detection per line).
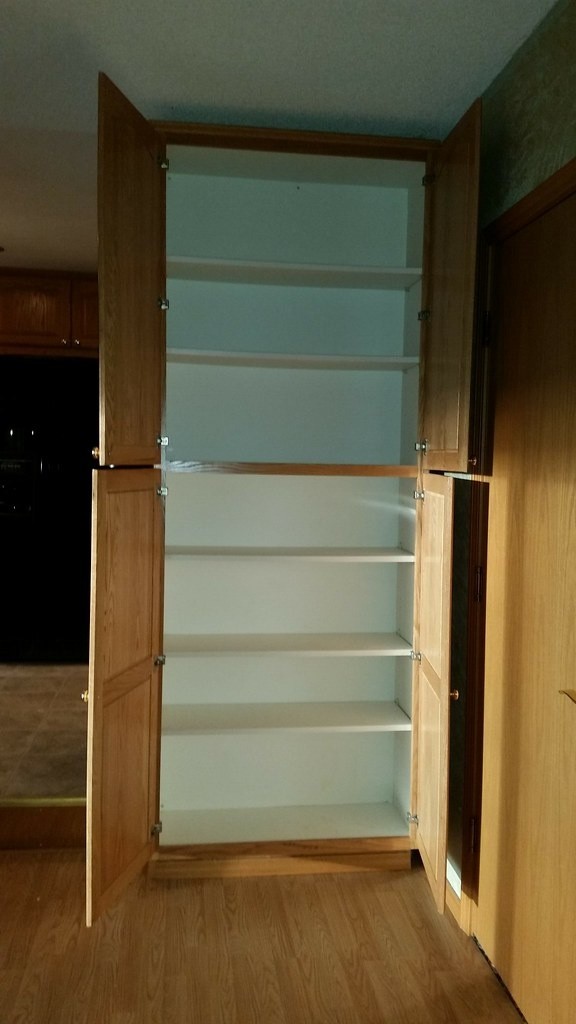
xmin=64 ymin=66 xmax=484 ymax=929
xmin=0 ymin=266 xmax=100 ymax=358
